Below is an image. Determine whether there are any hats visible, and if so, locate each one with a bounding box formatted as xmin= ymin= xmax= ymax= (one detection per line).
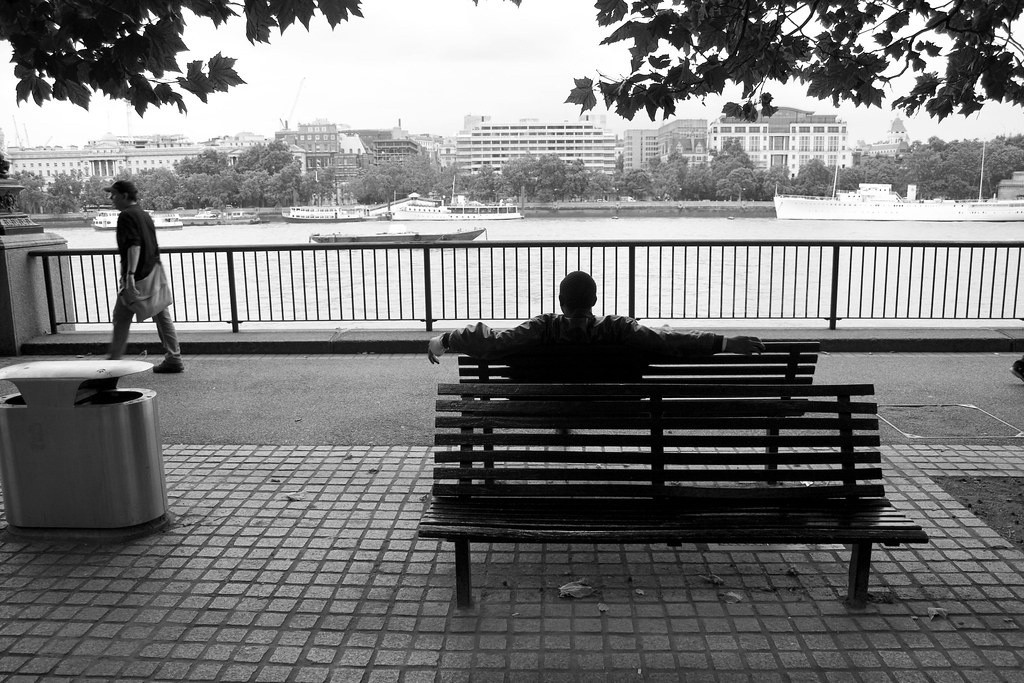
xmin=104 ymin=180 xmax=138 ymax=200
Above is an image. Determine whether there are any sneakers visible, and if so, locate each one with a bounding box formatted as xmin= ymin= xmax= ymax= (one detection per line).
xmin=153 ymin=359 xmax=184 ymax=374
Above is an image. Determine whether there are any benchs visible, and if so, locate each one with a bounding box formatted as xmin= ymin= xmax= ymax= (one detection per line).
xmin=458 ymin=338 xmax=821 ymax=485
xmin=417 ymin=382 xmax=928 ymax=609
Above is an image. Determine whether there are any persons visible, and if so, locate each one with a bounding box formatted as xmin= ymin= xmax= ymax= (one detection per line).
xmin=427 ymin=271 xmax=767 ymax=438
xmin=103 ymin=180 xmax=185 ymax=374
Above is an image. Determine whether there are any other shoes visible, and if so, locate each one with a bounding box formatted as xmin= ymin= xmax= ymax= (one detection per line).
xmin=1010 ymin=357 xmax=1024 ymax=382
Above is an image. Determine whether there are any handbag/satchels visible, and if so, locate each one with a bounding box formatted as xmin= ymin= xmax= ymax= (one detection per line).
xmin=120 ymin=260 xmax=172 ymax=321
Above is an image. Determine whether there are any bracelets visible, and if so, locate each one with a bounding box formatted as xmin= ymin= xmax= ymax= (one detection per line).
xmin=126 ymin=270 xmax=136 ymax=276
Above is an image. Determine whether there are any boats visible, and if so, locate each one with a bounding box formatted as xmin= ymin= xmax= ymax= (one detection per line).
xmin=91 ymin=176 xmax=528 ymax=230
xmin=308 ymin=227 xmax=487 ymax=244
xmin=772 ymin=142 xmax=1024 ymax=223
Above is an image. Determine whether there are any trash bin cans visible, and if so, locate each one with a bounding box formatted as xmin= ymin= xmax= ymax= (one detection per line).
xmin=0 ymin=360 xmax=170 ymax=541
